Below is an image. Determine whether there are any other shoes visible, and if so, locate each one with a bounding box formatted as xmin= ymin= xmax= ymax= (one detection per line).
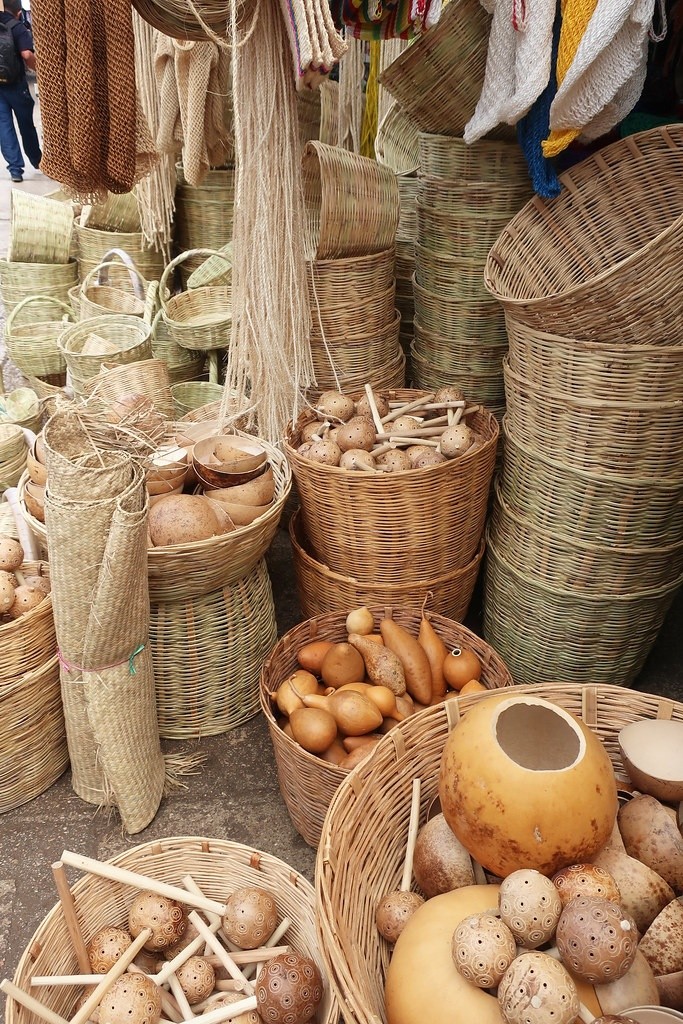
xmin=11 ymin=174 xmax=22 ymax=181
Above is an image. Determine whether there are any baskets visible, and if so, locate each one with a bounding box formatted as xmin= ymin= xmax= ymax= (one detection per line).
xmin=0 ymin=0 xmax=683 ymax=1024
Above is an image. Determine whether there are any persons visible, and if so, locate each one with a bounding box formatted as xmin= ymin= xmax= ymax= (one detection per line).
xmin=0 ymin=0 xmax=42 ymax=183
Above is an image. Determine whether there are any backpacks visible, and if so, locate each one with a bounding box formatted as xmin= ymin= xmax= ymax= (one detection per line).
xmin=0 ymin=18 xmax=24 ymax=85
